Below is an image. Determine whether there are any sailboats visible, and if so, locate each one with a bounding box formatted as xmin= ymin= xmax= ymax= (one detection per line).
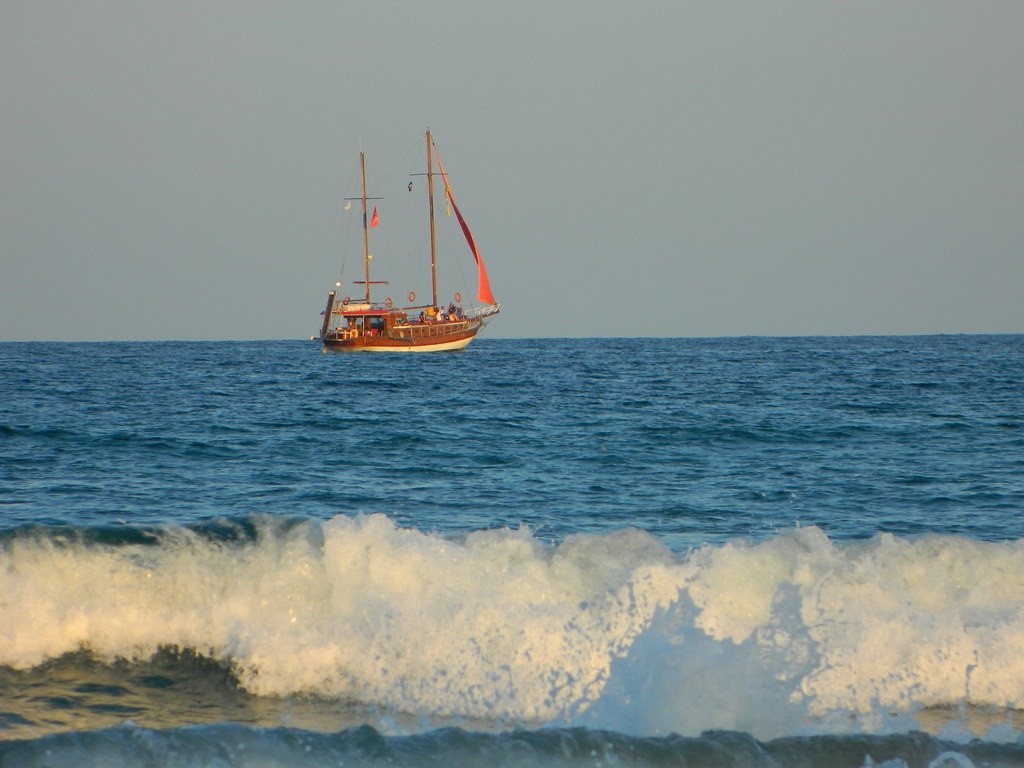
xmin=320 ymin=130 xmax=499 ymax=351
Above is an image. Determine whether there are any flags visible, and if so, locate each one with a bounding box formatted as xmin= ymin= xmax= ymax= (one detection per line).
xmin=369 ymin=199 xmax=379 ymax=230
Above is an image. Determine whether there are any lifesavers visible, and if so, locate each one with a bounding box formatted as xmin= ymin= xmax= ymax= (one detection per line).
xmin=349 ymin=329 xmax=358 ymax=338
xmin=386 ymin=297 xmax=393 ymax=308
xmin=455 ymin=292 xmax=462 ymax=303
xmin=408 ymin=291 xmax=415 ymax=302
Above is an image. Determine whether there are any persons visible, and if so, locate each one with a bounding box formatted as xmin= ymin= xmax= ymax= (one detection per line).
xmin=419 ymin=301 xmax=466 ymax=325
